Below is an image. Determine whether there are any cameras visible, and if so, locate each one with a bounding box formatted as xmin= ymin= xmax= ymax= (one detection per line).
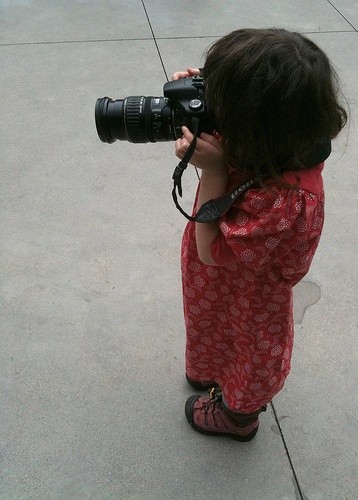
xmin=95 ymin=74 xmax=214 ymax=144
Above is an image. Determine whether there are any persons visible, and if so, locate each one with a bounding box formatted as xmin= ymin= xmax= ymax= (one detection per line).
xmin=172 ymin=29 xmax=348 ymax=442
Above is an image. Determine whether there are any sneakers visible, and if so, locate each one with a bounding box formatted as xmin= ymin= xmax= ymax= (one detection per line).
xmin=186 ymin=372 xmax=219 ymax=391
xmin=185 ymin=387 xmax=267 ymax=442
